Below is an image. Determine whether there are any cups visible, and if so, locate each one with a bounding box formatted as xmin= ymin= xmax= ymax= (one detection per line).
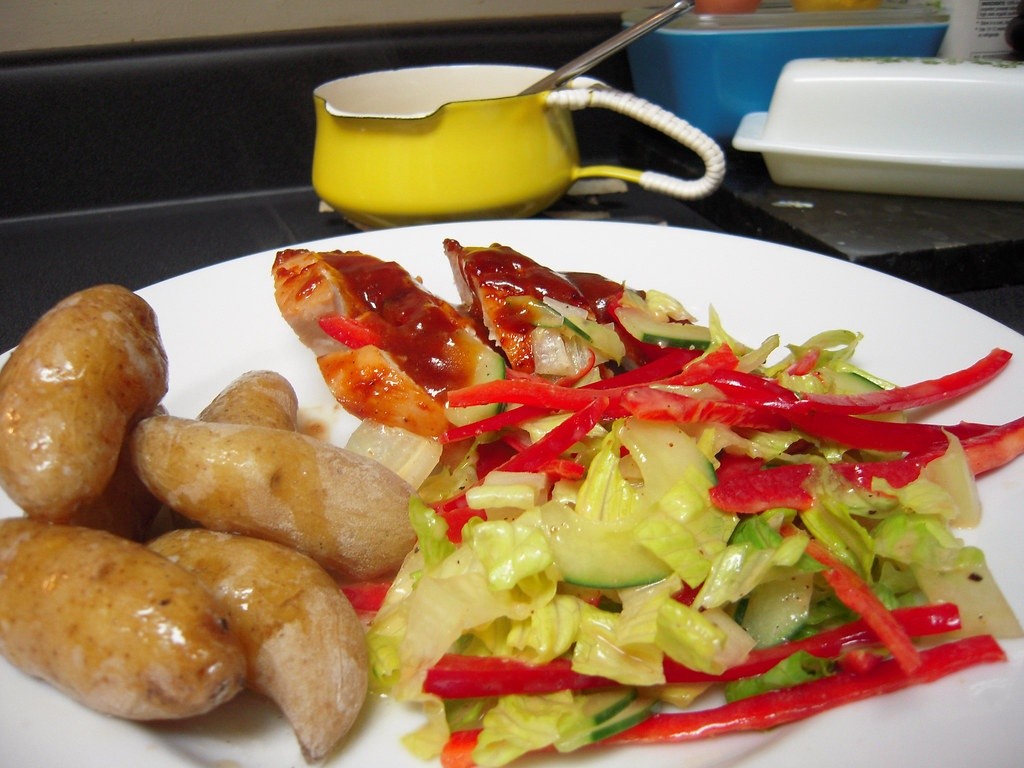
xmin=311 ymin=66 xmax=728 ymax=232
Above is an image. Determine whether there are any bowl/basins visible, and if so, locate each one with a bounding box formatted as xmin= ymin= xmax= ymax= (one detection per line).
xmin=622 ymin=5 xmax=950 ymax=144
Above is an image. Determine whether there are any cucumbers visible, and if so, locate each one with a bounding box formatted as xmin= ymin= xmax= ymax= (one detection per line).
xmin=452 ymin=291 xmax=904 ymax=753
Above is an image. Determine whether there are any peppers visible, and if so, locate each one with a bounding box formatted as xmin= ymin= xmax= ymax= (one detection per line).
xmin=318 ymin=314 xmax=1024 ymax=768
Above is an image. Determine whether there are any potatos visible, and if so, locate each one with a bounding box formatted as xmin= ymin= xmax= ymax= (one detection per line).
xmin=0 ymin=279 xmax=433 ymax=759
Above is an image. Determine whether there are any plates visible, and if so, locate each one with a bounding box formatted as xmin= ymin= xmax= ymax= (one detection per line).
xmin=730 ymin=111 xmax=1023 ymax=202
xmin=2 ymin=219 xmax=1022 ymax=768
